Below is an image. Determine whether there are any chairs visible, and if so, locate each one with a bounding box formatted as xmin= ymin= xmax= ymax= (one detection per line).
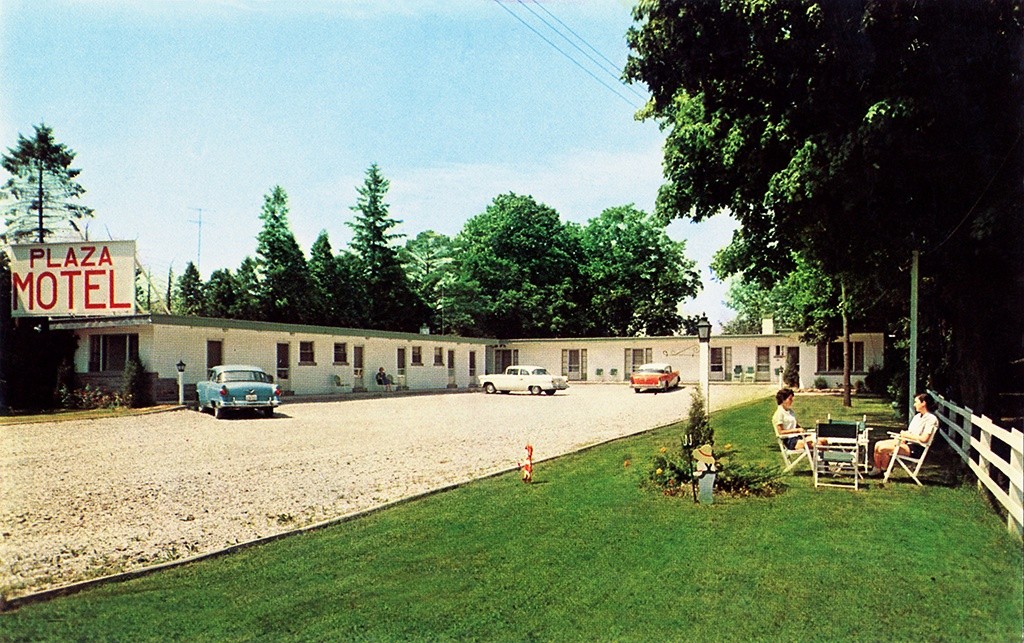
xmin=733 ymin=365 xmax=742 ymax=382
xmin=334 ymin=375 xmax=350 ymax=395
xmin=743 ymin=367 xmax=755 ymax=383
xmin=608 ymin=369 xmax=618 ymax=381
xmin=594 ymin=369 xmax=603 ymax=382
xmin=766 ymin=413 xmax=939 ymax=491
xmin=388 ymin=375 xmax=400 ymax=391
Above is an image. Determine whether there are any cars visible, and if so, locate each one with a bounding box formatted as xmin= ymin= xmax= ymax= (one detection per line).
xmin=195 ymin=365 xmax=283 ymax=419
xmin=630 ymin=363 xmax=680 ymax=393
xmin=478 ymin=365 xmax=570 ymax=396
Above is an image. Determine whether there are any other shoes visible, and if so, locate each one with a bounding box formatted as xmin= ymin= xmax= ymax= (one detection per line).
xmin=863 ymin=472 xmax=884 ymax=479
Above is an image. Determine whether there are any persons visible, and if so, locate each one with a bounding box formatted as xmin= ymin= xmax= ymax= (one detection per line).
xmin=772 ymin=389 xmax=829 ymax=452
xmin=375 ymin=367 xmax=393 ymax=392
xmin=868 ymin=391 xmax=939 ymax=476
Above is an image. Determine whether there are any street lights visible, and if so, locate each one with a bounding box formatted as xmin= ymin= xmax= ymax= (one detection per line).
xmin=697 ymin=311 xmax=712 ymax=421
